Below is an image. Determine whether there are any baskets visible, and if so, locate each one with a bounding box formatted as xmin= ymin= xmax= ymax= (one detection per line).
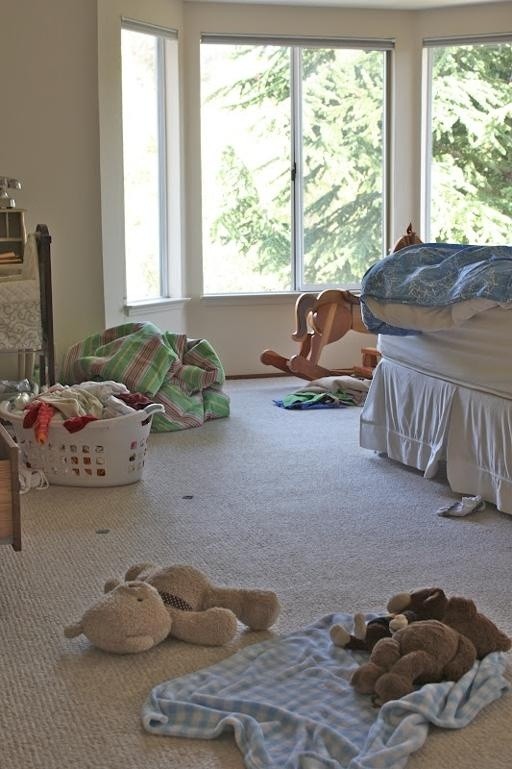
xmin=0 ymin=400 xmax=164 ymax=487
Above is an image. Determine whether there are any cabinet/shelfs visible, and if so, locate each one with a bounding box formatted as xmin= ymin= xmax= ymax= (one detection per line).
xmin=0 ymin=206 xmax=35 ymax=381
xmin=0 ymin=421 xmax=23 ymax=554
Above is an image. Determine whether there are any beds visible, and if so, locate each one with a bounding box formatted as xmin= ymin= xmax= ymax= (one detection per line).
xmin=0 ymin=223 xmax=57 ymax=387
xmin=359 ymin=243 xmax=512 ymax=518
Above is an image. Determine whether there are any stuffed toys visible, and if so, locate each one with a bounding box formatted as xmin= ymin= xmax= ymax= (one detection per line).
xmin=328 ymin=586 xmax=449 ymax=652
xmin=348 ymin=595 xmax=512 ymax=711
xmin=58 ymin=558 xmax=285 ymax=658
